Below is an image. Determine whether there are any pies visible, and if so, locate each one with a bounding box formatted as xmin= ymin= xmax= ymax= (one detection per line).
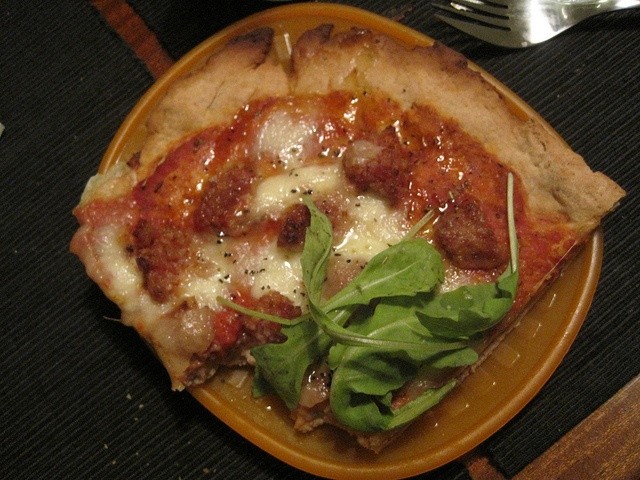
xmin=67 ymin=26 xmax=626 ymax=451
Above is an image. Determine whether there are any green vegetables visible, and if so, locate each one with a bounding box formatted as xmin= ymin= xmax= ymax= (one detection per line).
xmin=217 ymin=170 xmax=519 ymax=434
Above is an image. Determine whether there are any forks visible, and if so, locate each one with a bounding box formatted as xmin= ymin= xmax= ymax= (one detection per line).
xmin=432 ymin=0 xmax=640 ymax=49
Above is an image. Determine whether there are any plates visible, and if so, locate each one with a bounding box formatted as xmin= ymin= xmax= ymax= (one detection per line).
xmin=99 ymin=0 xmax=603 ymax=479
